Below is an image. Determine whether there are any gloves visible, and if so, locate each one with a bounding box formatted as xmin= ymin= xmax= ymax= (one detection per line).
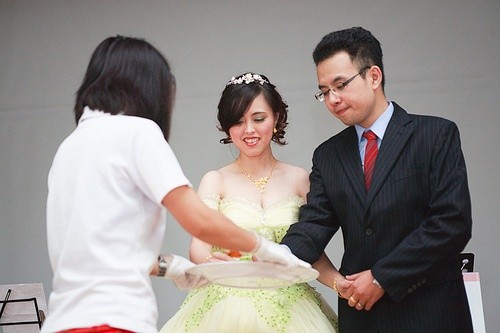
xmin=250 ymin=233 xmax=312 ymax=271
xmin=164 ymin=253 xmax=211 ymax=291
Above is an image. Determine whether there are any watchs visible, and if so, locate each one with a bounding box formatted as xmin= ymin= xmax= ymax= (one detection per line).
xmin=158 ymin=258 xmax=168 ymax=276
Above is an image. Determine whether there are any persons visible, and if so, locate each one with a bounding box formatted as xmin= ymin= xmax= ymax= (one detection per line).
xmin=278 ymin=26 xmax=473 ymax=333
xmin=159 ymin=72 xmax=354 ymax=333
xmin=38 ymin=34 xmax=312 ymax=333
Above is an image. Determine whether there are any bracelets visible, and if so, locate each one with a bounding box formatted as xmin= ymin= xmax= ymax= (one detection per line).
xmin=373 ymin=278 xmax=382 ymax=289
xmin=205 ymin=256 xmax=212 ymax=261
xmin=333 ymin=275 xmax=343 ymax=291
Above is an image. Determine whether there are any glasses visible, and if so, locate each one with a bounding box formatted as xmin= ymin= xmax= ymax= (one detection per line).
xmin=314 ymin=65 xmax=371 ymax=103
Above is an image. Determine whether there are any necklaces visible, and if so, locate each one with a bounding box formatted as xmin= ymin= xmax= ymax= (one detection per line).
xmin=237 ymin=159 xmax=276 ymax=193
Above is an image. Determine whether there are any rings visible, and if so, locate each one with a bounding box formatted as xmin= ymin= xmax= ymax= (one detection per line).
xmin=358 ymin=303 xmax=361 ymax=307
xmin=350 ymin=296 xmax=358 ymax=303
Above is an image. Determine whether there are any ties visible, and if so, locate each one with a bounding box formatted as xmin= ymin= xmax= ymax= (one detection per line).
xmin=361 ymin=131 xmax=379 ymax=194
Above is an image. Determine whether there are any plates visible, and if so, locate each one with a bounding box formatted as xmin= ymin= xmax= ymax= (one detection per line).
xmin=184 ymin=259 xmax=320 ymax=289
